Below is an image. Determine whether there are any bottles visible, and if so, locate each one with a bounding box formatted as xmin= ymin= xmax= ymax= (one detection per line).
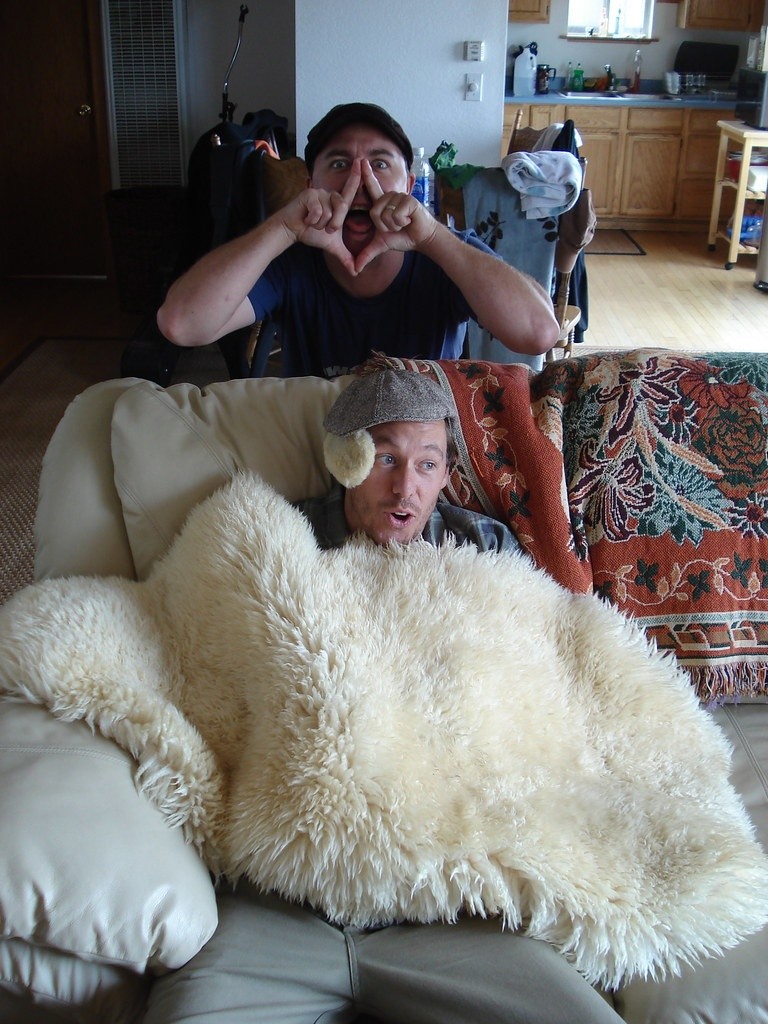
xmin=573 ymin=63 xmax=584 ymax=91
xmin=513 ymin=45 xmax=537 ymax=98
xmin=210 ymin=132 xmax=221 ymax=148
xmin=565 ymin=61 xmax=574 ymax=91
xmin=409 ymin=147 xmax=429 ymax=209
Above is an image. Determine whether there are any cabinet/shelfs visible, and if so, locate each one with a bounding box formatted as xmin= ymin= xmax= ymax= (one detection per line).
xmin=676 ymin=0 xmax=766 ymax=32
xmin=564 ymin=104 xmax=687 ymax=232
xmin=706 ymin=119 xmax=768 ymax=270
xmin=673 ymin=108 xmax=744 ymax=233
xmin=508 ymin=0 xmax=551 ymax=23
xmin=502 ymin=103 xmax=565 ymax=160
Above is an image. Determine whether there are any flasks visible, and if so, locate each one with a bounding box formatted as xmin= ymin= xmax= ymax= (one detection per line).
xmin=537 ymin=64 xmax=557 ymax=94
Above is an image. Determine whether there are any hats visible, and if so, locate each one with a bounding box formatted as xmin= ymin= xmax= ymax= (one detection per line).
xmin=323 ymin=369 xmax=457 ymax=437
xmin=556 ymin=189 xmax=596 ymax=273
xmin=305 ymin=103 xmax=412 ymax=177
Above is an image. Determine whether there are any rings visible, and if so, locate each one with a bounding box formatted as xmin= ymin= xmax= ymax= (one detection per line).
xmin=386 ymin=205 xmax=396 ymax=210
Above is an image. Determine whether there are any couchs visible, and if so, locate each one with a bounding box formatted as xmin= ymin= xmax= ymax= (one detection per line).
xmin=0 ymin=348 xmax=768 ymax=1024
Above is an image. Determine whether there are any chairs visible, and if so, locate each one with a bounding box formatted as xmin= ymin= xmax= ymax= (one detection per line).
xmin=507 ymin=108 xmax=547 ymax=155
xmin=434 ymin=156 xmax=588 ymax=363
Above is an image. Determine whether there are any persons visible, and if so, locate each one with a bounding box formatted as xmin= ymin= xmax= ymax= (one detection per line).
xmin=288 ymin=368 xmax=525 ymax=557
xmin=155 ymin=102 xmax=562 ymax=380
